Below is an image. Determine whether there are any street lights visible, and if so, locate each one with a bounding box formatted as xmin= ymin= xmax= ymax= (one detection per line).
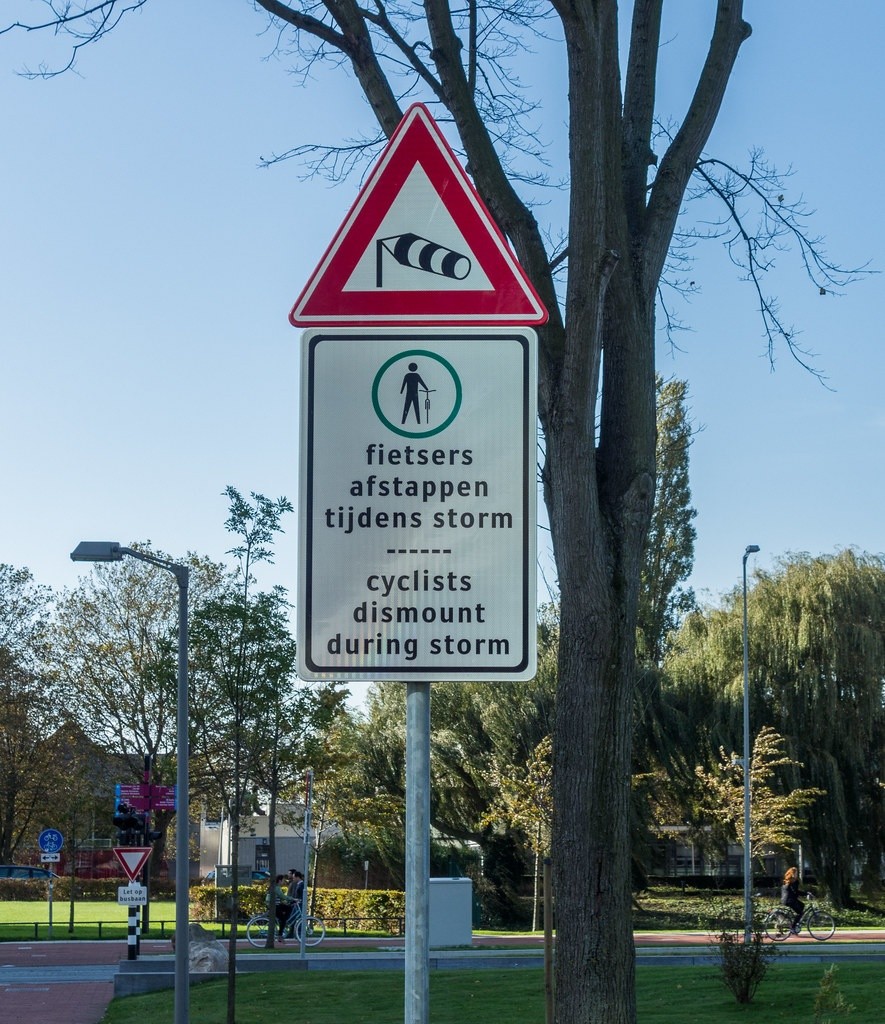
xmin=70 ymin=537 xmax=191 ymax=1024
xmin=738 ymin=543 xmax=764 ymax=944
xmin=299 ymin=765 xmax=316 ymax=958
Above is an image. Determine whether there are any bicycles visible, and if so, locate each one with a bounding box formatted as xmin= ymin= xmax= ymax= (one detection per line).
xmin=247 ymin=899 xmax=326 ymax=948
xmin=762 ymin=894 xmax=836 ymax=942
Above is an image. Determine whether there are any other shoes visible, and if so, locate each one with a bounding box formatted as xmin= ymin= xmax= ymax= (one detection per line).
xmin=277 ymin=936 xmax=286 ymax=944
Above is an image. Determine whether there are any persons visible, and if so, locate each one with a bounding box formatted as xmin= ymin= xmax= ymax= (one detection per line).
xmin=283 ymin=872 xmax=303 ymax=939
xmin=782 ymin=867 xmax=810 ymax=936
xmin=278 ymin=869 xmax=297 ymax=934
xmin=265 ymin=875 xmax=298 ymax=943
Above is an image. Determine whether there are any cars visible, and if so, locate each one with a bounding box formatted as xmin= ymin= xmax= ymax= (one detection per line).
xmin=0 ymin=865 xmax=58 ymax=880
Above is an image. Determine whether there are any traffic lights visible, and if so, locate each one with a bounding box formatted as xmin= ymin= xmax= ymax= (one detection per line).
xmin=111 ymin=802 xmax=132 ymax=848
xmin=139 ymin=812 xmax=163 ymax=848
